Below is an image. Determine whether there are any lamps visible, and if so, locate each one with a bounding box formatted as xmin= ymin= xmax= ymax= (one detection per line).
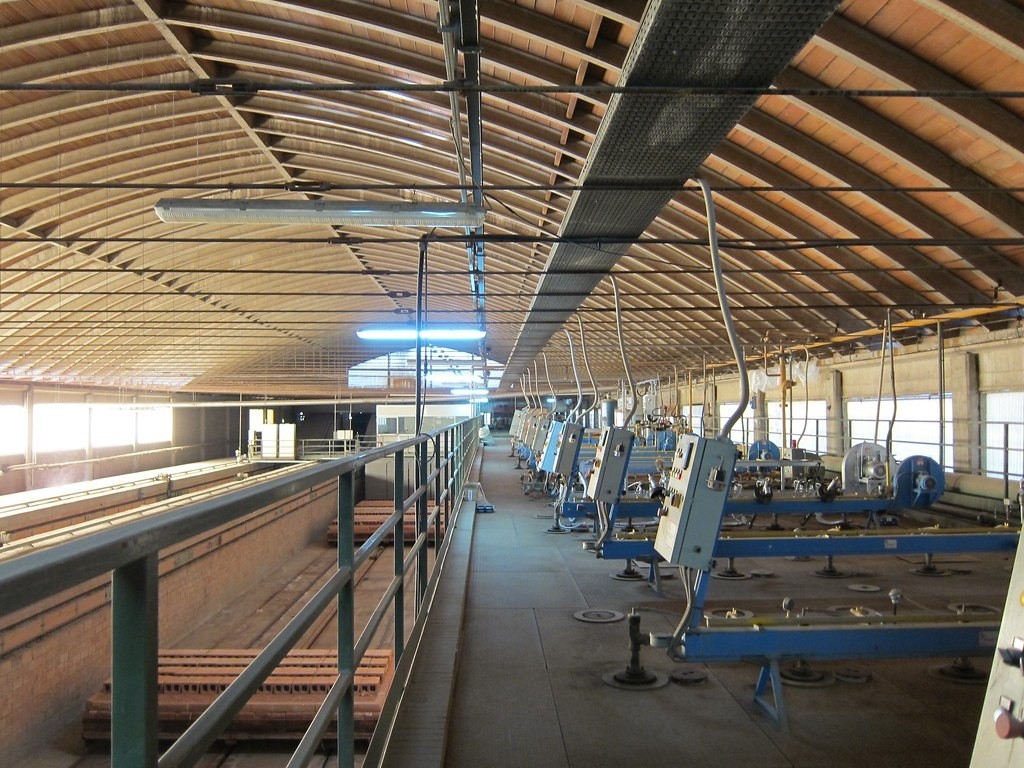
xmin=155 ymin=124 xmax=493 ymax=227
xmin=356 ymin=324 xmax=486 ymax=339
